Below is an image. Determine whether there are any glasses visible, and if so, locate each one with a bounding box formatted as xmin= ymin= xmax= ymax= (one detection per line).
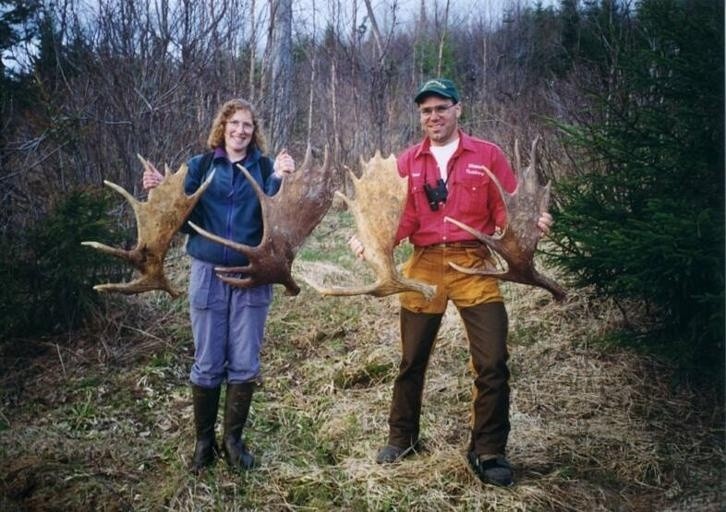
xmin=419 ymin=104 xmax=456 ymax=114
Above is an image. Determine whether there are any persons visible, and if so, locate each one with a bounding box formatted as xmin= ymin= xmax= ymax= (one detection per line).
xmin=346 ymin=80 xmax=554 ymax=485
xmin=143 ymin=99 xmax=296 ymax=474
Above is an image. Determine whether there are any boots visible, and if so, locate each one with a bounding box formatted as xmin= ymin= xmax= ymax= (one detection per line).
xmin=187 ymin=383 xmax=222 ymax=476
xmin=223 ymin=382 xmax=256 ymax=469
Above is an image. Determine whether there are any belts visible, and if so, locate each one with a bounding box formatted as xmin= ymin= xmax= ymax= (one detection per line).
xmin=424 ymin=240 xmax=482 ymax=248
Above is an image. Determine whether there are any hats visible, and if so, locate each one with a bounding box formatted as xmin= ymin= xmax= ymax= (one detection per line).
xmin=414 ymin=79 xmax=458 ymax=104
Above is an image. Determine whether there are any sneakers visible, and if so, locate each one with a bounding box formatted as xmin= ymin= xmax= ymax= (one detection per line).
xmin=375 ymin=444 xmax=421 ymax=464
xmin=467 ymin=453 xmax=514 ymax=488
